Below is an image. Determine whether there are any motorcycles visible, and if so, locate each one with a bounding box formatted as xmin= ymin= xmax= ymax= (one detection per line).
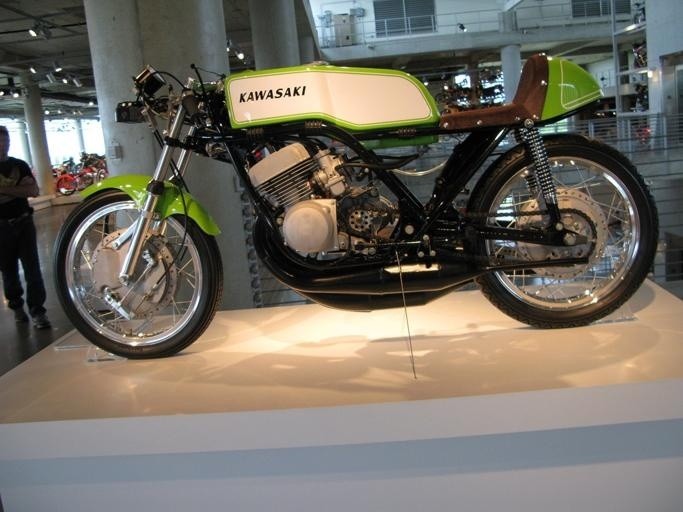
xmin=432 ymin=75 xmax=505 ymax=115
xmin=51 ymin=152 xmax=110 ymax=197
xmin=630 ymin=0 xmax=649 ymax=147
xmin=52 ymin=51 xmax=659 ymax=361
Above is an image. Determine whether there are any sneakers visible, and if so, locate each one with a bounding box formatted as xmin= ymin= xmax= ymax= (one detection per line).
xmin=15 ymin=311 xmax=51 ymax=328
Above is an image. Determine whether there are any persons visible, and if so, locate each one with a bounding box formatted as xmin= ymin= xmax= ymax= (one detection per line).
xmin=0 ymin=126 xmax=51 ymax=330
